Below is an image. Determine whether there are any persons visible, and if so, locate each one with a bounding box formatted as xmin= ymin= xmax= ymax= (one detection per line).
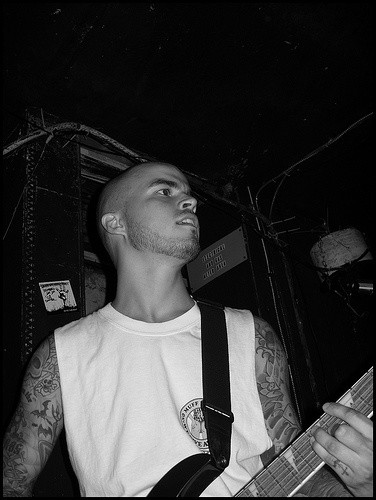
xmin=1 ymin=158 xmax=373 ymax=498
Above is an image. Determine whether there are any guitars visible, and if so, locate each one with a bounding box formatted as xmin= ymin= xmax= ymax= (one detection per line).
xmin=230 ymin=366 xmax=373 ymax=496
